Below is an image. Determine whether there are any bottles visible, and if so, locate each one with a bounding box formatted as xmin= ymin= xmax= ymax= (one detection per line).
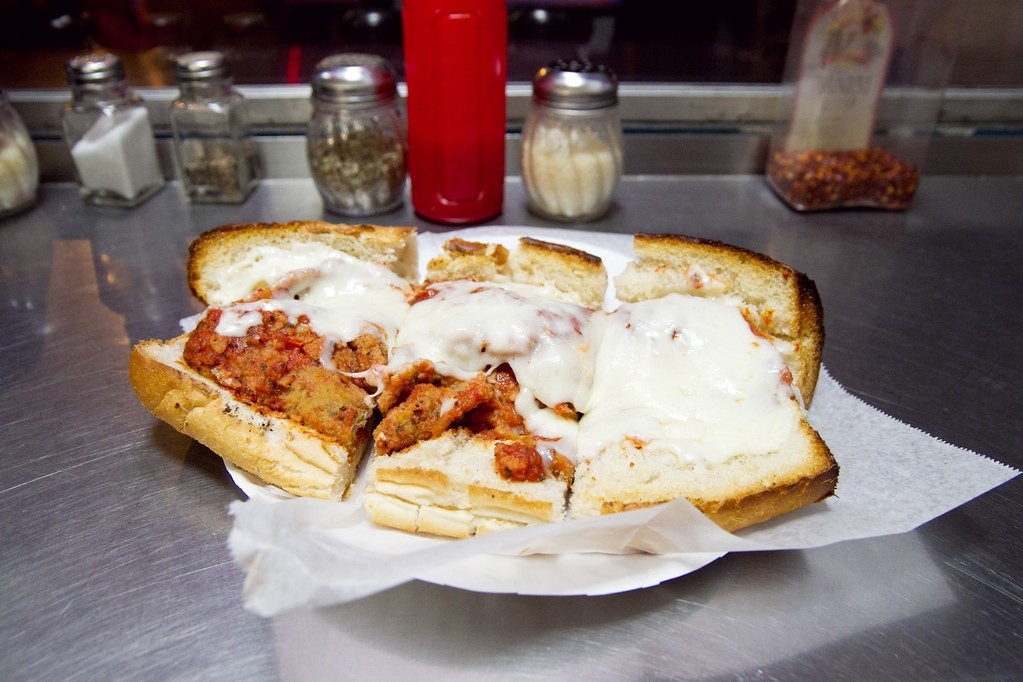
xmin=166 ymin=51 xmax=261 ymax=206
xmin=510 ymin=0 xmax=583 ymax=72
xmin=523 ymin=60 xmax=626 ymax=223
xmin=0 ymin=92 xmax=38 ymax=220
xmin=84 ymin=182 xmax=196 ymax=348
xmin=34 ymin=1 xmax=397 ymax=82
xmin=307 ymin=55 xmax=406 ymax=220
xmin=0 ymin=210 xmax=54 ymax=373
xmin=403 ymin=0 xmax=507 ymax=226
xmin=61 ymin=52 xmax=163 ymax=209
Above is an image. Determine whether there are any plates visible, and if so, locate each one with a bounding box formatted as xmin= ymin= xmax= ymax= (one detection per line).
xmin=226 ymin=214 xmax=728 ymax=620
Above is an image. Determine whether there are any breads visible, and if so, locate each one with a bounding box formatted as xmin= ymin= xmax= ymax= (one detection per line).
xmin=124 ymin=219 xmax=841 ymax=542
